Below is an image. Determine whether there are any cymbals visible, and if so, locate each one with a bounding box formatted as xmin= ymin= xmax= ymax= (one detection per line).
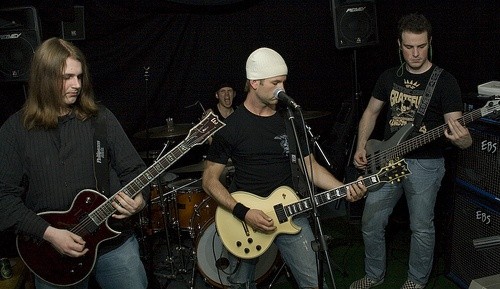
xmin=132 ymin=122 xmax=197 ymax=139
xmin=167 ymin=162 xmax=234 ymax=173
xmin=303 ymin=110 xmax=330 ymax=121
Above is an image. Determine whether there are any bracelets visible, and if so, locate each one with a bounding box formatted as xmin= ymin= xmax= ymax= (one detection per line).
xmin=232 ymin=203 xmax=250 ymax=221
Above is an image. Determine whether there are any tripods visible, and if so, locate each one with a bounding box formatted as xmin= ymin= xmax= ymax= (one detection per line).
xmin=148 ymin=176 xmax=202 ymax=289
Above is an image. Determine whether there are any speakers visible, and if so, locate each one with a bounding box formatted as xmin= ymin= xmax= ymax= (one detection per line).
xmin=330 ymin=0 xmax=380 ymax=49
xmin=0 ymin=7 xmax=44 ymax=82
xmin=442 ymin=115 xmax=500 ymax=289
xmin=61 ymin=6 xmax=85 ymax=41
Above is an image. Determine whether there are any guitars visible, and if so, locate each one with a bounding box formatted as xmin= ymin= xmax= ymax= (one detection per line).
xmin=214 ymin=159 xmax=412 ymax=260
xmin=15 ymin=108 xmax=227 ymax=289
xmin=350 ymin=95 xmax=500 ymax=193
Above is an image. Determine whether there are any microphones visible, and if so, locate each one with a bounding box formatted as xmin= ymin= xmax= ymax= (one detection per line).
xmin=272 ymin=88 xmax=300 ymax=109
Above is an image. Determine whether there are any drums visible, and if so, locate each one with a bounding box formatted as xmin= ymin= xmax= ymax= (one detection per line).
xmin=141 ymin=169 xmax=180 ymax=237
xmin=168 ymin=177 xmax=209 ymax=232
xmin=188 ymin=194 xmax=282 ymax=289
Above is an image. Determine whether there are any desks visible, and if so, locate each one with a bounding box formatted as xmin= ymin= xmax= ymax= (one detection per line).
xmin=0 ymin=257 xmax=35 ymax=289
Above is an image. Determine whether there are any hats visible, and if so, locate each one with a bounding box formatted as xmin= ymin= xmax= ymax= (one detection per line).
xmin=217 ymin=82 xmax=235 ymax=90
xmin=246 ymin=48 xmax=288 ymax=80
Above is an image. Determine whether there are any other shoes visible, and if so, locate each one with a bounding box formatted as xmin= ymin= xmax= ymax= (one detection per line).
xmin=400 ymin=279 xmax=426 ymax=289
xmin=350 ymin=276 xmax=385 ymax=289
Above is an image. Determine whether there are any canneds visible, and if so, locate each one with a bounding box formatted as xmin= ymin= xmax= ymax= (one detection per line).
xmin=0 ymin=258 xmax=13 ymax=280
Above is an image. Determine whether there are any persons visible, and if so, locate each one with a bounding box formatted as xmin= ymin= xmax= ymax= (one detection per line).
xmin=201 ymin=47 xmax=367 ymax=289
xmin=350 ymin=14 xmax=472 ymax=289
xmin=0 ymin=37 xmax=153 ymax=289
xmin=203 ymin=81 xmax=240 ymax=142
